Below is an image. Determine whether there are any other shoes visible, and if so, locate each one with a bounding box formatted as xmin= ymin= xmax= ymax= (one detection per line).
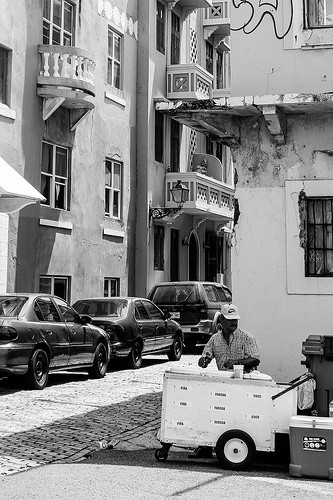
xmin=188 ymin=446 xmax=213 ymax=459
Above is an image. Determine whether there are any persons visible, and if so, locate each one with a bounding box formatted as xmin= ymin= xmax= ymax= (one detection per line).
xmin=198 ymin=304 xmax=261 ymax=373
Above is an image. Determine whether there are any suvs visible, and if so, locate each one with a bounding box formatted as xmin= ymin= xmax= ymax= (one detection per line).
xmin=147 ymin=281 xmax=233 ymax=346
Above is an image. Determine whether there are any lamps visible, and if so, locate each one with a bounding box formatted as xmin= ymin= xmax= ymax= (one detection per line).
xmin=170 ymin=180 xmax=189 ymax=207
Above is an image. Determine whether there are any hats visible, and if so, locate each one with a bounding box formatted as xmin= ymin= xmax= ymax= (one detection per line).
xmin=221 ymin=303 xmax=240 ymax=320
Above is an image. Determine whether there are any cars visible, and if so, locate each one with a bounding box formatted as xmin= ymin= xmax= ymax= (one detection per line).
xmin=71 ymin=297 xmax=183 ymax=368
xmin=1 ymin=294 xmax=112 ymax=390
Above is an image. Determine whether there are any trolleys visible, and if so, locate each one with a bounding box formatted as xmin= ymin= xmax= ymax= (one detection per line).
xmin=155 ymin=368 xmax=315 ymax=471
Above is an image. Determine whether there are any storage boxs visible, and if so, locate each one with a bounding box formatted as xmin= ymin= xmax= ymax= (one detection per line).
xmin=288 ymin=415 xmax=333 ymax=481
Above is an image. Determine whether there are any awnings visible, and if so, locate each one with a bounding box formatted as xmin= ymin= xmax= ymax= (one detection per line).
xmin=0 ymin=148 xmax=47 ymax=216
